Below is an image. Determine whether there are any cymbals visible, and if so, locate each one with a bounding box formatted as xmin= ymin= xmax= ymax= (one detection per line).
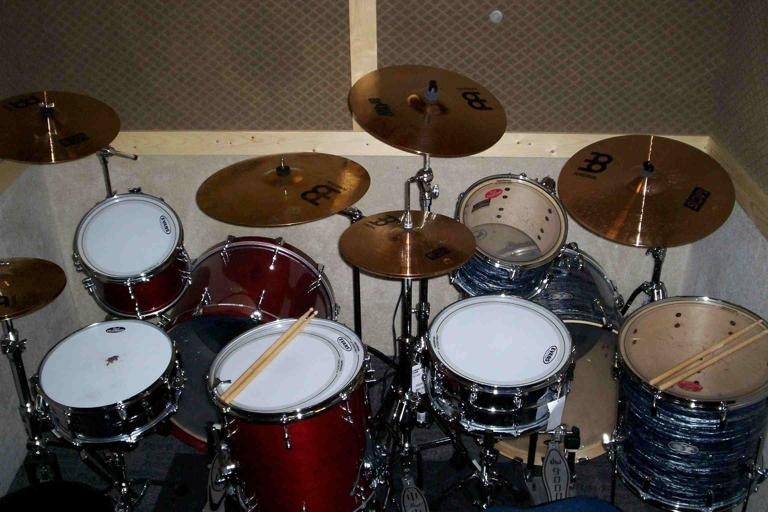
xmin=1 ymin=90 xmax=120 ymax=164
xmin=195 ymin=153 xmax=370 ymax=228
xmin=338 ymin=209 xmax=475 ymax=281
xmin=347 ymin=64 xmax=508 ymax=158
xmin=2 ymin=257 xmax=67 ymax=321
xmin=557 ymin=136 xmax=735 ymax=248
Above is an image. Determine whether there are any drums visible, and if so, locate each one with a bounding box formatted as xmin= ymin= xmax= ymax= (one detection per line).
xmin=208 ymin=318 xmax=373 ymax=511
xmin=422 ymin=294 xmax=573 ymax=437
xmin=73 ymin=192 xmax=191 ymax=319
xmin=609 ymin=295 xmax=768 ymax=512
xmin=156 ymin=235 xmax=340 ymax=449
xmin=453 ymin=173 xmax=567 ymax=298
xmin=460 ymin=244 xmax=626 ymax=468
xmin=31 ymin=320 xmax=184 ymax=446
